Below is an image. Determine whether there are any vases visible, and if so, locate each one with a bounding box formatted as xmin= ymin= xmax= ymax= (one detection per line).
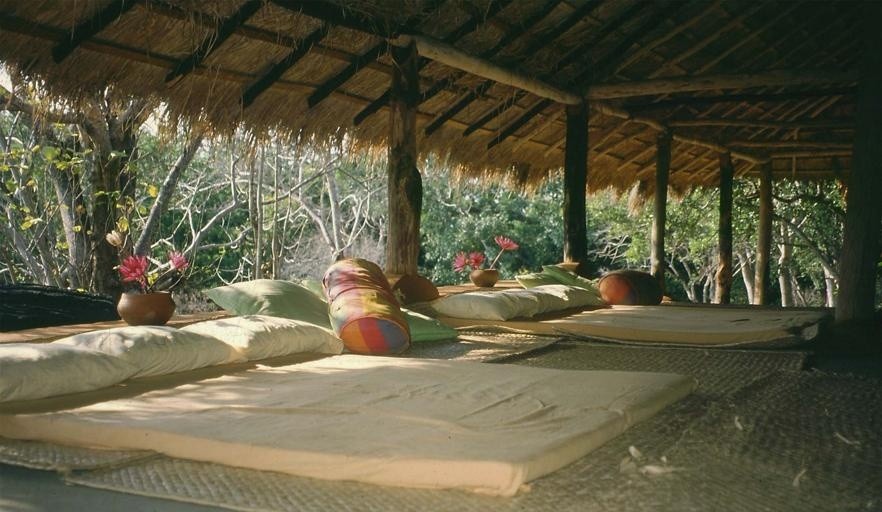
xmin=116 ymin=288 xmax=174 ymax=327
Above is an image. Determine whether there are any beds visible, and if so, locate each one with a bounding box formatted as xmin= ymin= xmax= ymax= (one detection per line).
xmin=0 ymin=301 xmax=836 ymax=496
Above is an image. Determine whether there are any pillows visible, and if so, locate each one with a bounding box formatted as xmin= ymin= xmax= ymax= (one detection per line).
xmin=430 ymin=267 xmax=663 ymax=321
xmin=201 ymin=262 xmax=452 ymax=355
xmin=0 ymin=322 xmax=241 ymax=403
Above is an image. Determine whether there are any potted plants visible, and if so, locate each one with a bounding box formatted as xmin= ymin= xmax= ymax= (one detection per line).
xmin=454 ymin=237 xmax=518 ymax=271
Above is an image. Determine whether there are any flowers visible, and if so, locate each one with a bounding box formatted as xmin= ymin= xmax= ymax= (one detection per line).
xmin=114 ymin=249 xmax=188 ymax=294
